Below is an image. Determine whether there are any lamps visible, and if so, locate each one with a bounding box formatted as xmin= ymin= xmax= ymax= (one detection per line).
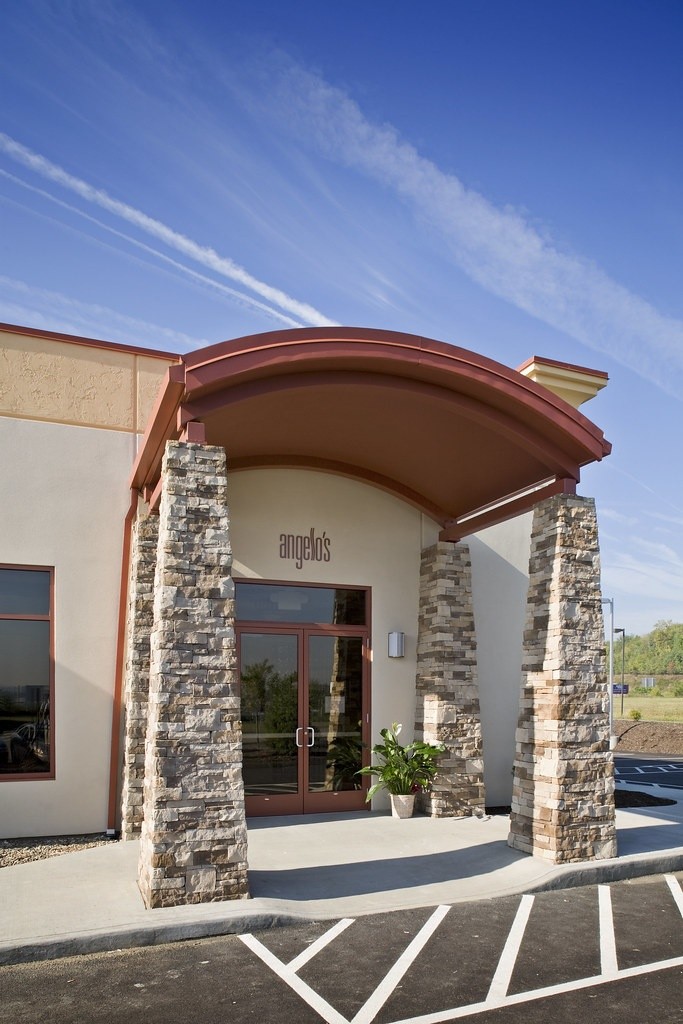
xmin=387 ymin=631 xmax=405 ymax=657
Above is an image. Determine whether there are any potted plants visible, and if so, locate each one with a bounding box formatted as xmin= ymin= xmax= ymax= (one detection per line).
xmin=353 ymin=719 xmax=449 ymax=821
xmin=326 ymin=734 xmax=362 ymax=791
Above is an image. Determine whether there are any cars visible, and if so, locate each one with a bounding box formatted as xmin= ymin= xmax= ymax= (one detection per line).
xmin=613 ymin=682 xmax=627 ymax=694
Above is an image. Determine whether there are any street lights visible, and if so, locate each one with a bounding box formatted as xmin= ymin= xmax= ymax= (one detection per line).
xmin=602 ymin=597 xmax=620 ymax=752
xmin=613 ymin=626 xmax=626 ymax=716
